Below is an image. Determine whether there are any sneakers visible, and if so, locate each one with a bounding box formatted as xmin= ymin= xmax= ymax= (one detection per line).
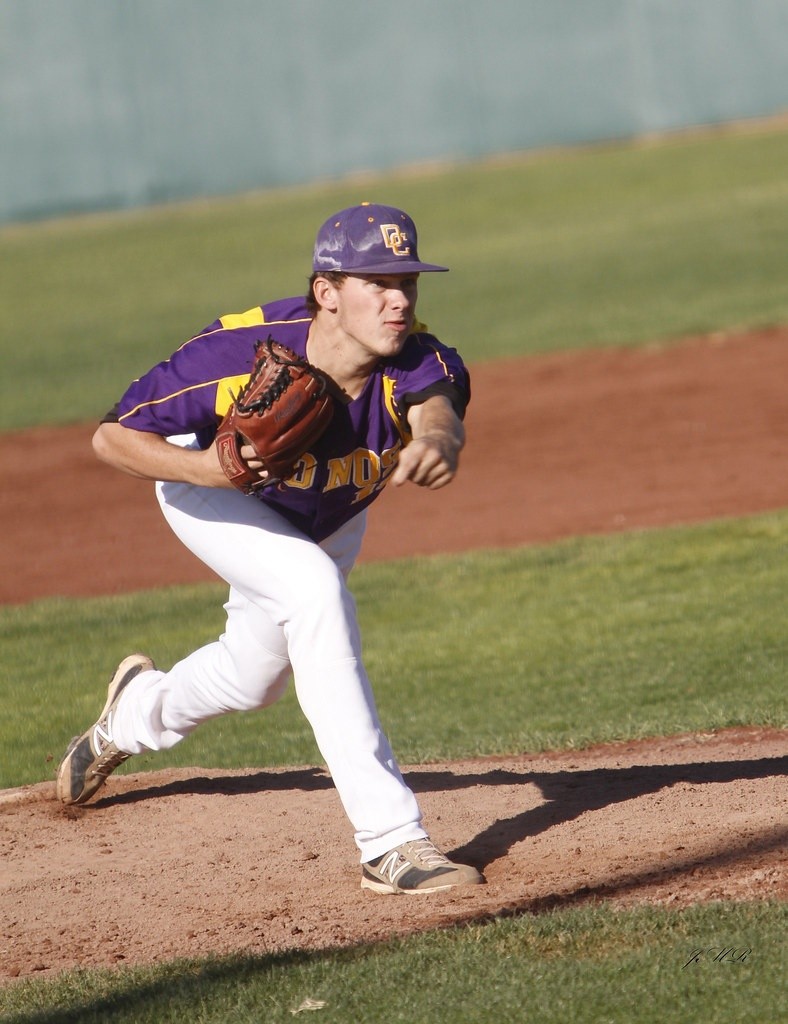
xmin=361 ymin=838 xmax=484 ymax=895
xmin=55 ymin=652 xmax=156 ymax=805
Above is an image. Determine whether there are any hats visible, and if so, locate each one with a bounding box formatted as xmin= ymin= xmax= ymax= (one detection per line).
xmin=314 ymin=202 xmax=449 ymax=274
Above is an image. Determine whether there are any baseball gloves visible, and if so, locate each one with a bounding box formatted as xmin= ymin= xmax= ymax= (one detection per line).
xmin=214 ymin=337 xmax=350 ymax=497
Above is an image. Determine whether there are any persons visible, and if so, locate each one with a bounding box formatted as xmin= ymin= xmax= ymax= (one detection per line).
xmin=55 ymin=203 xmax=484 ymax=894
